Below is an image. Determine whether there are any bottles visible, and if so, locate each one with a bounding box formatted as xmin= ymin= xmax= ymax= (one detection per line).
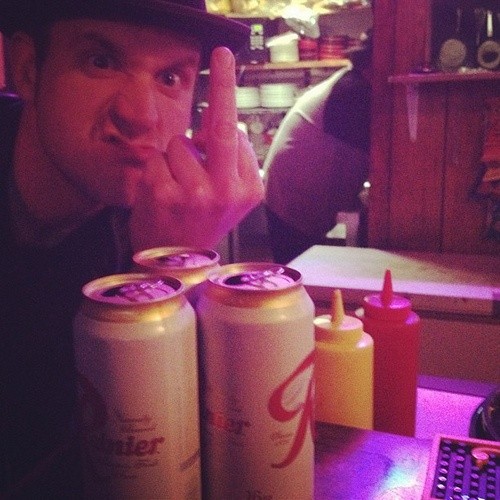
xmin=354 ymin=268 xmax=421 ymax=438
xmin=314 ymin=289 xmax=374 ymax=430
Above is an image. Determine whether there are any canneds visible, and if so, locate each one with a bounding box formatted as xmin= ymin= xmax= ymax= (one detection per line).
xmin=73 ymin=274 xmax=202 ymax=500
xmin=193 ymin=262 xmax=315 ymax=500
xmin=127 ymin=247 xmax=220 ymax=305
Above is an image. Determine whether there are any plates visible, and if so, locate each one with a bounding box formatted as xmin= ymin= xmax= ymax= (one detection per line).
xmin=235 ymin=81 xmax=311 ymax=109
xmin=265 ymin=32 xmax=365 ymax=63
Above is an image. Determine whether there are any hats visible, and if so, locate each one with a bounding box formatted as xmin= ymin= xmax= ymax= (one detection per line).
xmin=0 ymin=0 xmax=251 ymax=71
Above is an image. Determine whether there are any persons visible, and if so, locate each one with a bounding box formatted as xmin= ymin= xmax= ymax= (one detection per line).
xmin=0 ymin=0 xmax=265 ymax=500
xmin=260 ymin=28 xmax=374 ymax=266
